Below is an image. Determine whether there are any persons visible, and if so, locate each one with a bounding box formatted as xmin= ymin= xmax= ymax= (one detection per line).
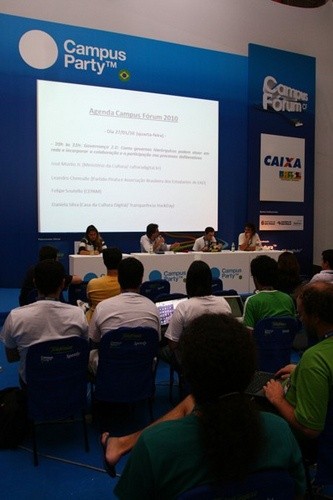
xmin=19 ymin=245 xmax=82 ymax=306
xmin=309 ymin=249 xmax=333 ymax=285
xmin=161 ymin=261 xmax=232 ymax=364
xmin=191 ymin=227 xmax=222 ymax=251
xmin=76 ymin=248 xmax=123 ymax=324
xmin=277 ymin=252 xmax=302 ymax=293
xmin=87 ymin=258 xmax=161 ymax=377
xmin=241 ymin=255 xmax=296 ymax=332
xmin=78 ymin=225 xmax=107 ymax=255
xmin=0 ymin=260 xmax=89 ymax=393
xmin=140 ymin=224 xmax=179 ymax=253
xmin=97 ymin=312 xmax=308 ymax=500
xmin=238 ymin=222 xmax=262 ymax=251
xmin=263 ymin=279 xmax=333 ymax=461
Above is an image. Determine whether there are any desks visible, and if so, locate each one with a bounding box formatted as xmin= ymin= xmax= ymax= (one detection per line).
xmin=68 ymin=249 xmax=304 ymax=295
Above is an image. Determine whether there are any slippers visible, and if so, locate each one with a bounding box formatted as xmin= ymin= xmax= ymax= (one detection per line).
xmin=99 ymin=431 xmax=117 ymax=478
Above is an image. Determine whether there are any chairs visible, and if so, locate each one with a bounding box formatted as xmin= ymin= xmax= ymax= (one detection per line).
xmin=18 ymin=278 xmax=333 ymax=500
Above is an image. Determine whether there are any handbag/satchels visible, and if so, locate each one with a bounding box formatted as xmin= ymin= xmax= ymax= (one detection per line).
xmin=0 ymin=387 xmax=26 ymax=450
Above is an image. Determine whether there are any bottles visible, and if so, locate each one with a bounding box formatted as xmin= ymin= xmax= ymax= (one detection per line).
xmin=255 ymin=240 xmax=259 ymax=251
xmin=148 ymin=243 xmax=153 ymax=254
xmin=231 ymin=242 xmax=235 ymax=251
xmin=90 ymin=245 xmax=94 ymax=255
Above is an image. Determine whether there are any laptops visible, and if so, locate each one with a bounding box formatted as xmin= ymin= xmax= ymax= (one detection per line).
xmin=244 ymin=371 xmax=286 ymax=398
xmin=154 ymin=296 xmax=188 ymax=327
xmin=223 ymin=296 xmax=245 ymax=322
